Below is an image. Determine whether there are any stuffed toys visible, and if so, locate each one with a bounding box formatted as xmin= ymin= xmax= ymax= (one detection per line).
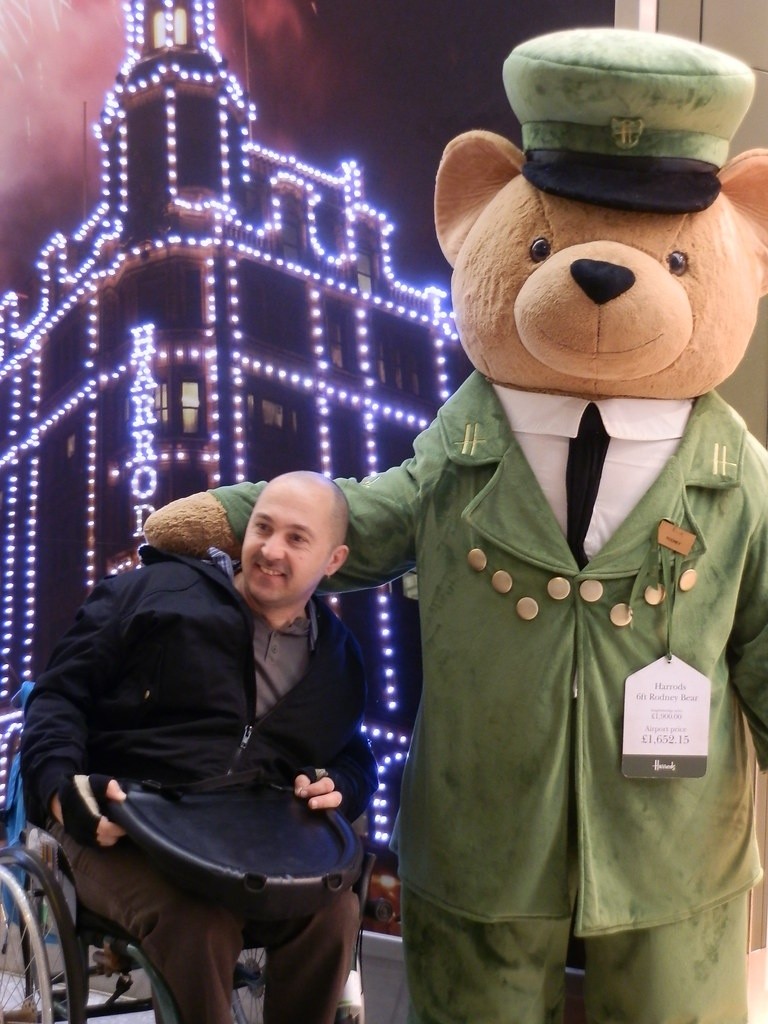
xmin=142 ymin=27 xmax=768 ymax=1024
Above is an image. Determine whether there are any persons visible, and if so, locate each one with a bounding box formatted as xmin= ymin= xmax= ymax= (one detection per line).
xmin=19 ymin=470 xmax=380 ymax=1024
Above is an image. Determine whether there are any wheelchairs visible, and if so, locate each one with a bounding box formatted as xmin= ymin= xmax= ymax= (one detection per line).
xmin=0 ymin=682 xmax=365 ymax=1024
